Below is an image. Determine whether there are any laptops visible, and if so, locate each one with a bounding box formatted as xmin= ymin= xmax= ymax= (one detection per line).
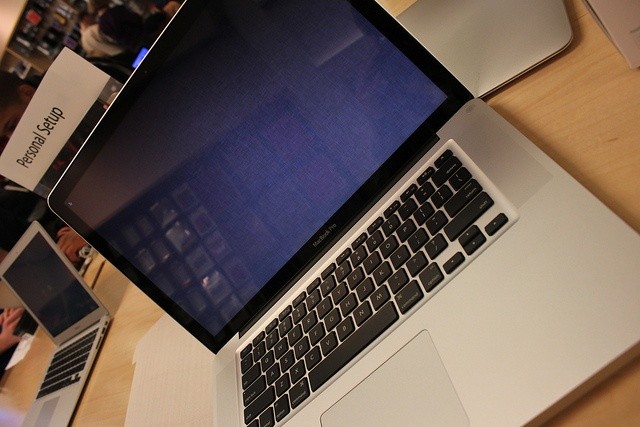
xmin=44 ymin=1 xmax=640 ymax=427
xmin=390 ymin=1 xmax=577 ymax=103
xmin=2 ymin=219 xmax=117 ymax=427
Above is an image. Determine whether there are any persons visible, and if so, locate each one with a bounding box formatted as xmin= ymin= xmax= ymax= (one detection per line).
xmin=0 ymin=67 xmax=105 ymax=263
xmin=78 ymin=0 xmax=180 ymax=60
xmin=0 ymin=306 xmax=26 ymax=354
